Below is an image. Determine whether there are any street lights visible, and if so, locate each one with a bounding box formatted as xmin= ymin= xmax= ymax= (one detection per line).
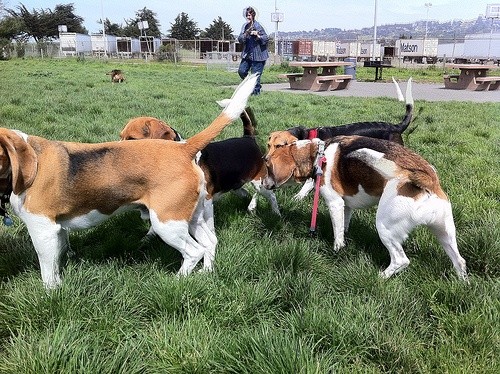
xmin=424 ymin=2 xmax=433 ymax=40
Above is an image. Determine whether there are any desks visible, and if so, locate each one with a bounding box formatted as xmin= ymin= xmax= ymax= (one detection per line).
xmin=445 ymin=63 xmax=498 ymax=90
xmin=288 ymin=62 xmax=354 ymax=90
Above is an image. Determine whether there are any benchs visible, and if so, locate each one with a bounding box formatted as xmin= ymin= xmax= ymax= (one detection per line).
xmin=443 ymin=75 xmax=460 ymax=89
xmin=278 ymin=73 xmax=322 ymax=90
xmin=475 ymin=76 xmax=500 ymax=91
xmin=316 ymin=75 xmax=354 ymax=91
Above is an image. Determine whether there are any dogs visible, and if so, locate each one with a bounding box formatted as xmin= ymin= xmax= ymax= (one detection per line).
xmin=1 ymin=68 xmax=260 ymax=293
xmin=265 ymin=75 xmax=416 ymax=202
xmin=106 ymin=69 xmax=126 ymax=84
xmin=120 ymin=99 xmax=284 ymax=243
xmin=260 ymin=134 xmax=470 ymax=288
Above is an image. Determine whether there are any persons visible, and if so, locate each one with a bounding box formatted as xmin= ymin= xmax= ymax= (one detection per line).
xmin=238 ymin=7 xmax=269 ymax=95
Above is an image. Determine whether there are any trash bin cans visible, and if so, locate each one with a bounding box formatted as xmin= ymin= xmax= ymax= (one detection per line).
xmin=344 ymin=57 xmax=357 ymax=79
